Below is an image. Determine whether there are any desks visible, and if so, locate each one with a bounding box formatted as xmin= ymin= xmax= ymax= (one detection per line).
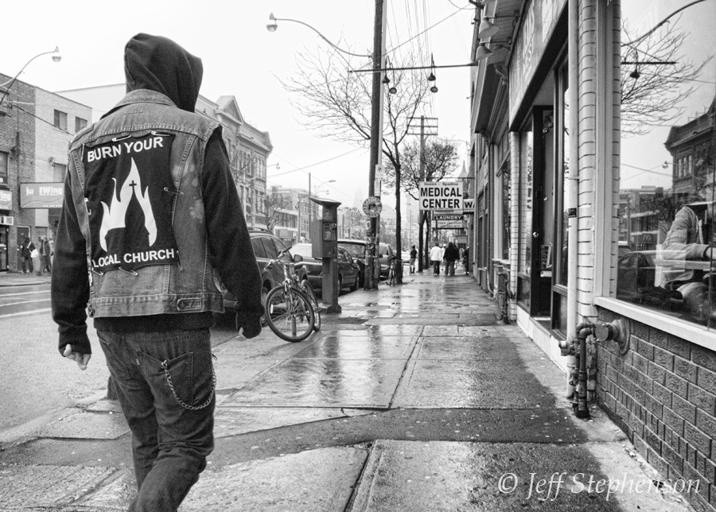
xmin=656 ymin=259 xmax=716 ymax=294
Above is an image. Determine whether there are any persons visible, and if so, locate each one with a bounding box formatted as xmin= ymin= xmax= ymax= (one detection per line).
xmin=51 ymin=33 xmax=265 ymax=512
xmin=429 ymin=242 xmax=469 ymax=276
xmin=662 ymin=164 xmax=716 ymax=319
xmin=21 ymin=235 xmax=51 ymax=274
xmin=409 ymin=246 xmax=417 ymax=273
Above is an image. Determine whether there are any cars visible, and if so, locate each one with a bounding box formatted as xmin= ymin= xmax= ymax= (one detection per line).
xmin=287 ymin=242 xmax=362 ymax=296
xmin=336 ymin=237 xmax=397 ymax=282
xmin=216 ymin=230 xmax=306 ymax=323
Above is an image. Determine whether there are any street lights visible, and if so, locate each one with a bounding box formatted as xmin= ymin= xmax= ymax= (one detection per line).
xmin=265 ymin=13 xmax=382 ymax=292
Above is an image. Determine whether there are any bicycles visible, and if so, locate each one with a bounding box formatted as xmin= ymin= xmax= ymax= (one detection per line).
xmin=258 ymin=244 xmax=323 ymax=344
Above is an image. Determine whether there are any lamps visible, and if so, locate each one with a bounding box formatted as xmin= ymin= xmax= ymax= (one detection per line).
xmin=474 ymin=14 xmax=519 ymax=62
xmin=383 ymin=54 xmax=439 ymax=98
